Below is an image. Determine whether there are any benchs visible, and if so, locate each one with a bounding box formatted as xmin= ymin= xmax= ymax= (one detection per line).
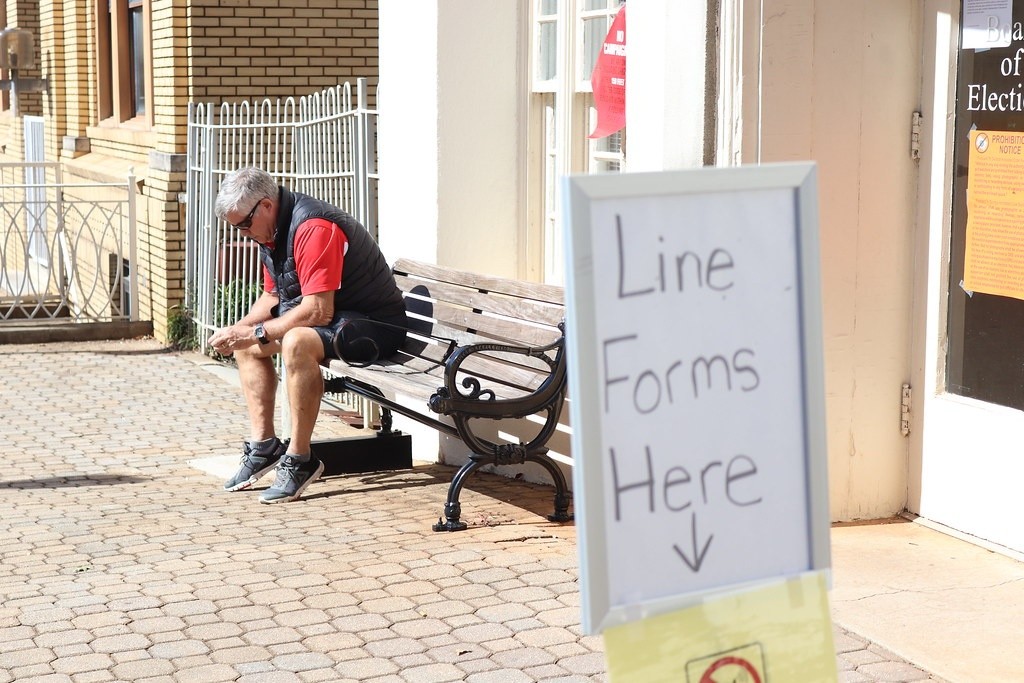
xmin=318 ymin=257 xmax=571 ymax=532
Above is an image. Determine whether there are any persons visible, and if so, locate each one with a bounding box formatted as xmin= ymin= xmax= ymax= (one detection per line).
xmin=207 ymin=167 xmax=408 ymax=504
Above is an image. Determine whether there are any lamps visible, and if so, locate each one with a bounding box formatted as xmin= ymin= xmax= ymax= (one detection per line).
xmin=0 ymin=27 xmax=50 ymax=94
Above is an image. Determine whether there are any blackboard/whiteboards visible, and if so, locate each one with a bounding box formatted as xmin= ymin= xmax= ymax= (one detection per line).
xmin=563 ymin=158 xmax=835 ymax=634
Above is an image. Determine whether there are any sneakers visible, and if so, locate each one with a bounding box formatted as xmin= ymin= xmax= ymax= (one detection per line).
xmin=259 ymin=448 xmax=324 ymax=503
xmin=223 ymin=436 xmax=287 ymax=491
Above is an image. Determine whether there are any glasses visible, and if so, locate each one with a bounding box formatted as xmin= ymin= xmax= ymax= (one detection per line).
xmin=231 ymin=199 xmax=262 ymax=231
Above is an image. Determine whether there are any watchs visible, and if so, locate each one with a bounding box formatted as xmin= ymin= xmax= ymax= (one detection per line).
xmin=254 ymin=323 xmax=270 ymax=345
